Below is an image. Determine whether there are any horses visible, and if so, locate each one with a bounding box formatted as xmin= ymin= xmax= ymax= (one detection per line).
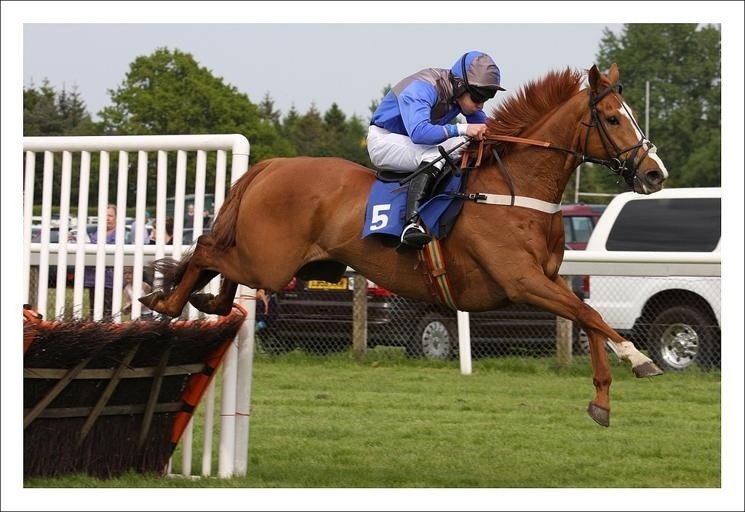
xmin=137 ymin=63 xmax=670 ymax=427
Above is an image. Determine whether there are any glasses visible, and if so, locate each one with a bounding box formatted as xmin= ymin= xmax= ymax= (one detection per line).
xmin=469 ymin=91 xmax=489 ymax=104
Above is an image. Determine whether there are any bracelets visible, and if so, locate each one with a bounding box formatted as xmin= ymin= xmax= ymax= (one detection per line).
xmin=459 ymin=124 xmax=470 ymax=135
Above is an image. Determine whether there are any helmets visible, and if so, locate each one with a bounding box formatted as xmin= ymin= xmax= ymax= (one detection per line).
xmin=450 ymin=51 xmax=505 ymax=92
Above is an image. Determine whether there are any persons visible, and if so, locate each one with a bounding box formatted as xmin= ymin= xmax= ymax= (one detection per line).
xmin=33 ymin=200 xmax=213 ymax=320
xmin=365 ymin=51 xmax=506 ymax=252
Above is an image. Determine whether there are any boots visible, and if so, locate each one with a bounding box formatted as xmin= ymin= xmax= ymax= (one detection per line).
xmin=394 ymin=160 xmax=441 ymax=255
